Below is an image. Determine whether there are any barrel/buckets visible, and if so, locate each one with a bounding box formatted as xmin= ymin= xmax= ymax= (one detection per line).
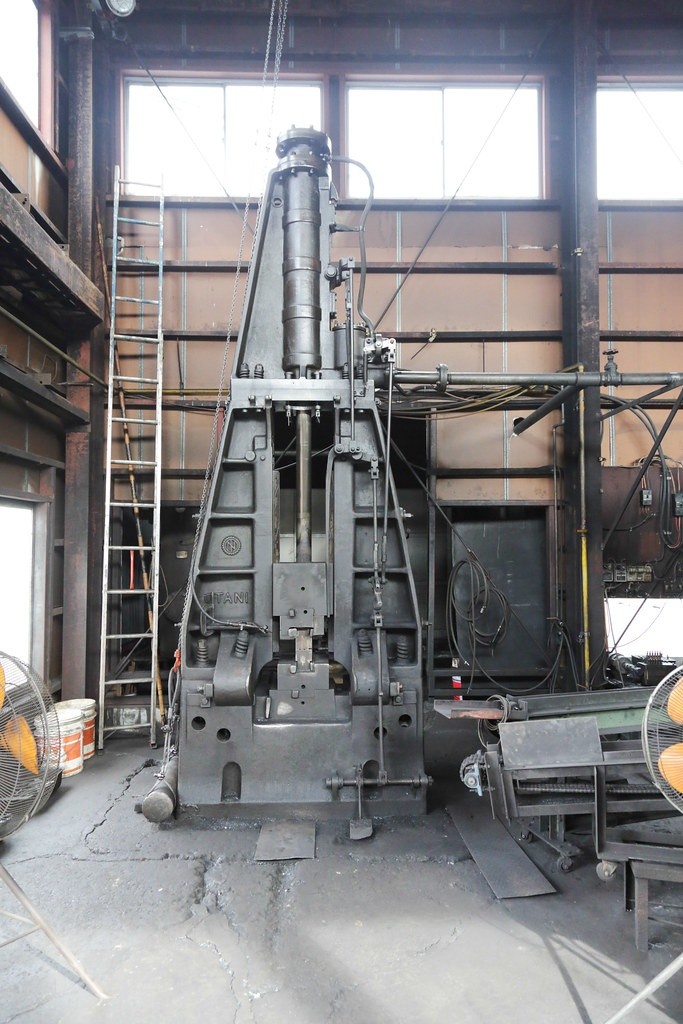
xmin=49 ymin=698 xmax=97 ymax=760
xmin=34 ymin=710 xmax=85 ymax=778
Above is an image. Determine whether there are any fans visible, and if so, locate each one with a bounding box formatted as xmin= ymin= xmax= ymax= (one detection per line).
xmin=0 ymin=650 xmax=61 ymax=839
xmin=641 ymin=665 xmax=683 ymax=816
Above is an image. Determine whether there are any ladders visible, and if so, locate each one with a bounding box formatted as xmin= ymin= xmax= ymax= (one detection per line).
xmin=98 ymin=164 xmax=166 ymax=752
